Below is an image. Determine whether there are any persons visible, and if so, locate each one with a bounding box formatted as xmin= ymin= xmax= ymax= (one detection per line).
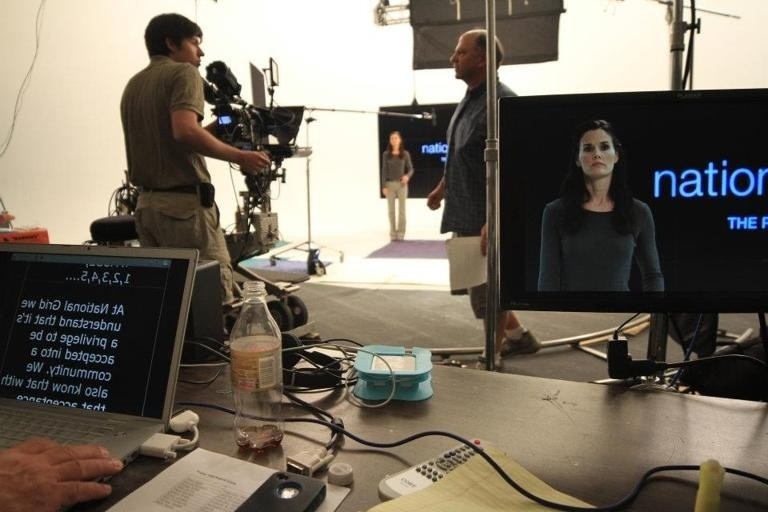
xmin=538 ymin=118 xmax=664 ymax=293
xmin=1 ymin=435 xmax=125 ymax=512
xmin=118 ymin=12 xmax=272 ymax=336
xmin=424 ymin=27 xmax=544 ymax=373
xmin=381 ymin=130 xmax=414 ymax=241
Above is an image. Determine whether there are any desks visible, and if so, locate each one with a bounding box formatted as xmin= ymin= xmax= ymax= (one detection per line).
xmin=75 ymin=348 xmax=768 ymax=512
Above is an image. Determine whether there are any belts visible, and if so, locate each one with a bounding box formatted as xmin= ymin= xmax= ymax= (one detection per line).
xmin=143 ymin=185 xmax=199 ymax=194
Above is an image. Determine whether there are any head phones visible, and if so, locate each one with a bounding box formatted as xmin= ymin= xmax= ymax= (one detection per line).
xmin=281 ymin=330 xmax=342 ymax=388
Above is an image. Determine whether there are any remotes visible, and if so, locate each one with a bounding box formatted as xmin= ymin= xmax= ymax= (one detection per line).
xmin=375 ymin=436 xmax=493 ymax=502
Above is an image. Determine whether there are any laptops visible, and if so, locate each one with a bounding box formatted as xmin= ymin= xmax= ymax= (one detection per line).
xmin=0 ymin=243 xmax=199 ymax=512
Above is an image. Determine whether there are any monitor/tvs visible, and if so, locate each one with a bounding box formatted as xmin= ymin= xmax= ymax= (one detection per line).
xmin=497 ymin=88 xmax=768 ymax=314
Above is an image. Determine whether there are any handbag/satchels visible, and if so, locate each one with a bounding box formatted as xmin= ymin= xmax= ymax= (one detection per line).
xmin=200 ymin=182 xmax=215 ymax=208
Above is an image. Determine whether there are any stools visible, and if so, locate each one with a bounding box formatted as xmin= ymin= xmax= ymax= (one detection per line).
xmin=90 ymin=216 xmax=137 ymax=246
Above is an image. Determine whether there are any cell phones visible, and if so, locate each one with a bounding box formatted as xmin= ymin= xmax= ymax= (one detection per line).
xmin=233 ymin=471 xmax=326 ymax=512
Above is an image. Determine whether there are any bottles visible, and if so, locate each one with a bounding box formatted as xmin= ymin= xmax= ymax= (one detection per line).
xmin=231 ymin=280 xmax=285 ymax=452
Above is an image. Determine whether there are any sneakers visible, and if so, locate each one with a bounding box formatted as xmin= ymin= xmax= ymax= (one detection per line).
xmin=389 ymin=231 xmax=405 ymax=240
xmin=475 ymin=329 xmax=541 ymax=371
